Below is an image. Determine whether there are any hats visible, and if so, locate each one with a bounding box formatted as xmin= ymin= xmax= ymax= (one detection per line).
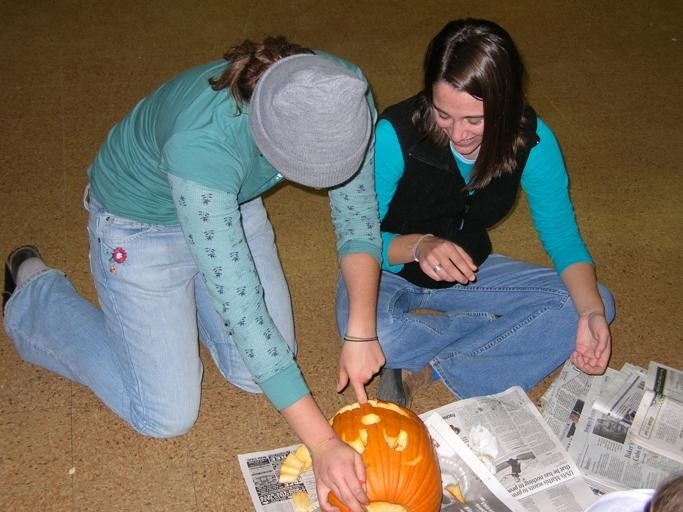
xmin=247 ymin=52 xmax=373 ymax=190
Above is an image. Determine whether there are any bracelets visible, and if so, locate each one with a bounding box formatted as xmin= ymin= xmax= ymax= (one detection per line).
xmin=433 ymin=263 xmax=443 ymax=273
xmin=343 ymin=334 xmax=379 ymax=343
xmin=413 ymin=234 xmax=435 ymax=262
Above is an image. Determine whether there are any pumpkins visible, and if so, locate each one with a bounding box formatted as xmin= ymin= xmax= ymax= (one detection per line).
xmin=276 ymin=400 xmax=443 ymax=512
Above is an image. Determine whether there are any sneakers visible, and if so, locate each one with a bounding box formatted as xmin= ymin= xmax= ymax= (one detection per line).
xmin=3 ymin=245 xmax=42 ymax=319
xmin=376 ymin=363 xmax=435 ymax=410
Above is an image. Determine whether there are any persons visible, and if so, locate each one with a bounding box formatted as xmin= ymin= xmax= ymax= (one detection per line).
xmin=3 ymin=36 xmax=386 ymax=512
xmin=336 ymin=17 xmax=615 ymax=410
